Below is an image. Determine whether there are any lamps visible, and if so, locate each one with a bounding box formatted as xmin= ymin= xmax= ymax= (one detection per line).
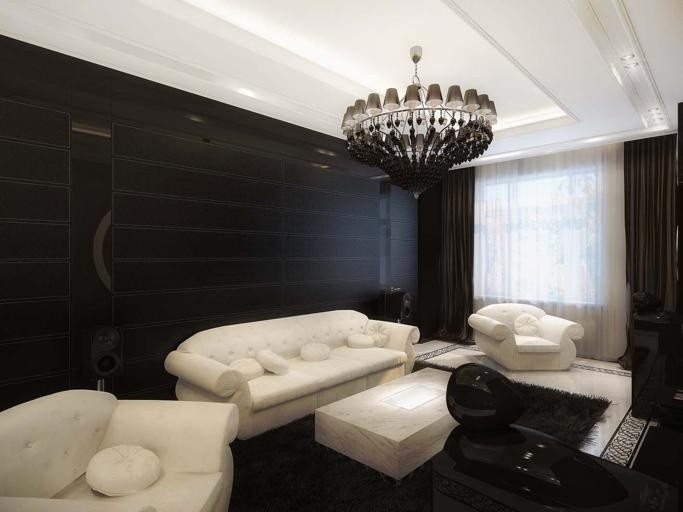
xmin=338 ymin=46 xmax=496 ymax=207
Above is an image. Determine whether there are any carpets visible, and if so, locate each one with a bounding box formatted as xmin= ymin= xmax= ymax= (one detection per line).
xmin=219 ymin=361 xmax=611 ymax=512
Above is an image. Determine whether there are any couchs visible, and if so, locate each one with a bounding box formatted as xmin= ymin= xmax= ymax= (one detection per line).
xmin=164 ymin=308 xmax=420 ymax=440
xmin=468 ymin=302 xmax=583 ymax=370
xmin=0 ymin=390 xmax=237 ymax=512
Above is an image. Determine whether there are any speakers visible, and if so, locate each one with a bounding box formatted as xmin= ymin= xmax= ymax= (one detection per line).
xmin=388 ymin=291 xmax=412 ymax=325
xmin=80 ymin=324 xmax=123 ymax=379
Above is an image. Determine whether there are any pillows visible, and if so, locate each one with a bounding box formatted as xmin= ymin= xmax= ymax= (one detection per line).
xmin=515 ymin=314 xmax=538 ymax=336
xmin=84 ymin=444 xmax=161 ymax=497
xmin=345 ymin=333 xmax=372 ymax=347
xmin=256 ymin=351 xmax=288 ymax=374
xmin=299 ymin=342 xmax=330 ymax=362
xmin=230 ymin=358 xmax=263 ymax=379
